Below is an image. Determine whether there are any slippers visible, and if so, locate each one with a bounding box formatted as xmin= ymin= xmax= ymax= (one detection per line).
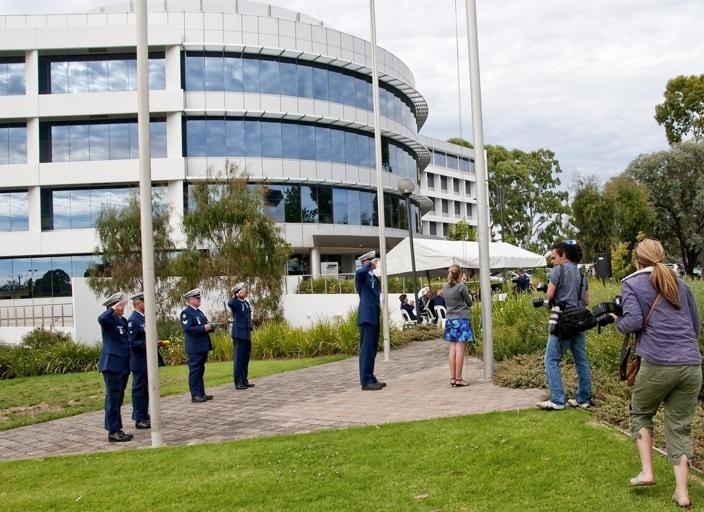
xmin=671 ymin=493 xmax=692 ymax=509
xmin=628 ymin=474 xmax=657 ymax=488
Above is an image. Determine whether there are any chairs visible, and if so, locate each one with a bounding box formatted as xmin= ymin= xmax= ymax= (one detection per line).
xmin=434 ymin=306 xmax=448 ymax=328
xmin=390 ymin=309 xmax=417 ymax=332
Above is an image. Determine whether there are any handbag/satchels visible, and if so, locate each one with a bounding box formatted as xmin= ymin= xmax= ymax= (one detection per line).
xmin=556 ymin=270 xmax=596 ymax=341
xmin=624 ymin=291 xmax=664 ymax=386
xmin=617 ymin=343 xmax=633 ymax=383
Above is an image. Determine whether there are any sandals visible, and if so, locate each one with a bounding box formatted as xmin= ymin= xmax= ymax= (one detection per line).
xmin=450 ymin=377 xmax=456 ymax=387
xmin=455 ymin=379 xmax=469 ymax=387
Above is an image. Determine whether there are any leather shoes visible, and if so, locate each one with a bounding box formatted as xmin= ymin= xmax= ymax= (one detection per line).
xmin=361 ymin=383 xmax=382 ymax=391
xmin=236 ymin=384 xmax=247 ymax=389
xmin=203 ymin=394 xmax=214 ymax=400
xmin=377 ymin=381 xmax=387 ymax=387
xmin=108 ymin=430 xmax=133 ymax=442
xmin=191 ymin=396 xmax=208 ymax=402
xmin=136 ymin=419 xmax=151 ymax=429
xmin=245 ymin=383 xmax=255 ymax=387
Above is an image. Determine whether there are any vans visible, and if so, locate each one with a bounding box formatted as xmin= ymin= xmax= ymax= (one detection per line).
xmin=525 ymin=265 xmax=553 ymax=283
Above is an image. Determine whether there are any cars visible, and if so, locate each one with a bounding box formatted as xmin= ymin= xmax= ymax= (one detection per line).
xmin=665 ymin=263 xmax=685 ymax=279
xmin=489 ymin=269 xmax=534 ymax=286
xmin=692 ymin=266 xmax=702 ymax=280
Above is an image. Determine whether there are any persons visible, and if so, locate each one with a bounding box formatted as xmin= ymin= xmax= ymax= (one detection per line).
xmin=355 ymin=250 xmax=386 ymax=390
xmin=536 ymin=243 xmax=592 ymax=410
xmin=128 ymin=293 xmax=170 ymax=430
xmin=606 ymin=236 xmax=703 ymax=509
xmin=514 ymin=268 xmax=532 ymax=296
xmin=180 ymin=288 xmax=215 ymax=402
xmin=227 ymin=282 xmax=254 ymax=390
xmin=442 ymin=265 xmax=474 ymax=387
xmin=569 ymin=243 xmax=584 ymax=267
xmin=399 ymin=294 xmax=417 ymax=323
xmin=98 ymin=292 xmax=134 ymax=442
xmin=435 ymin=289 xmax=447 ymax=326
xmin=417 ymin=286 xmax=436 ymax=322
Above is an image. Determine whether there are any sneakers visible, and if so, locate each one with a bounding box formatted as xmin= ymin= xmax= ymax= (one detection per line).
xmin=568 ymin=398 xmax=591 ymax=410
xmin=535 ymin=400 xmax=567 ymax=410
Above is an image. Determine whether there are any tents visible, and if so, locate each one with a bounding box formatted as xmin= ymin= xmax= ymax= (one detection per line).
xmin=371 ymin=229 xmax=550 ymax=277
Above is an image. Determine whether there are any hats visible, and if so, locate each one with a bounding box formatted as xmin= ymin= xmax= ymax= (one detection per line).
xmin=183 ymin=289 xmax=202 ymax=299
xmin=358 ymin=251 xmax=376 ymax=261
xmin=130 ymin=293 xmax=144 ymax=300
xmin=102 ymin=292 xmax=126 ymax=307
xmin=231 ymin=283 xmax=244 ymax=293
